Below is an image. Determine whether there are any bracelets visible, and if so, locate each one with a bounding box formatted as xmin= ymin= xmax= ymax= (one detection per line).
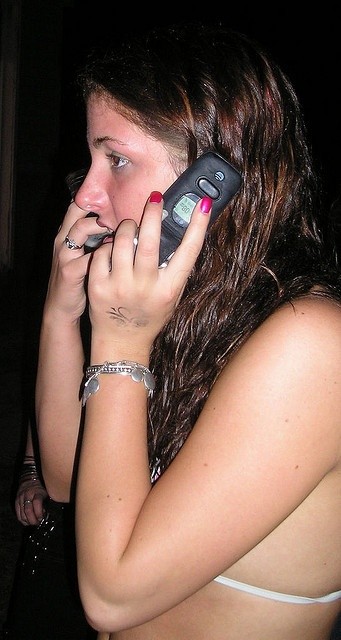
xmin=82 ymin=360 xmax=156 ymax=406
xmin=19 ymin=455 xmax=41 ymax=479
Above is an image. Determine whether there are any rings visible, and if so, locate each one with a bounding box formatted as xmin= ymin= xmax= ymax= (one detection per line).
xmin=64 ymin=237 xmax=83 ymax=250
xmin=24 ymin=500 xmax=33 ymax=504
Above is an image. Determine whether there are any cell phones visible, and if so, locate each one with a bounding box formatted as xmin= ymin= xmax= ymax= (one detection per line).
xmin=103 ymin=150 xmax=244 ymax=275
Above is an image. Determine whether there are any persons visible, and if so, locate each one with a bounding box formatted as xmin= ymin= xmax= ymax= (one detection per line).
xmin=35 ymin=20 xmax=341 ymax=640
xmin=15 ymin=420 xmax=47 ymax=526
